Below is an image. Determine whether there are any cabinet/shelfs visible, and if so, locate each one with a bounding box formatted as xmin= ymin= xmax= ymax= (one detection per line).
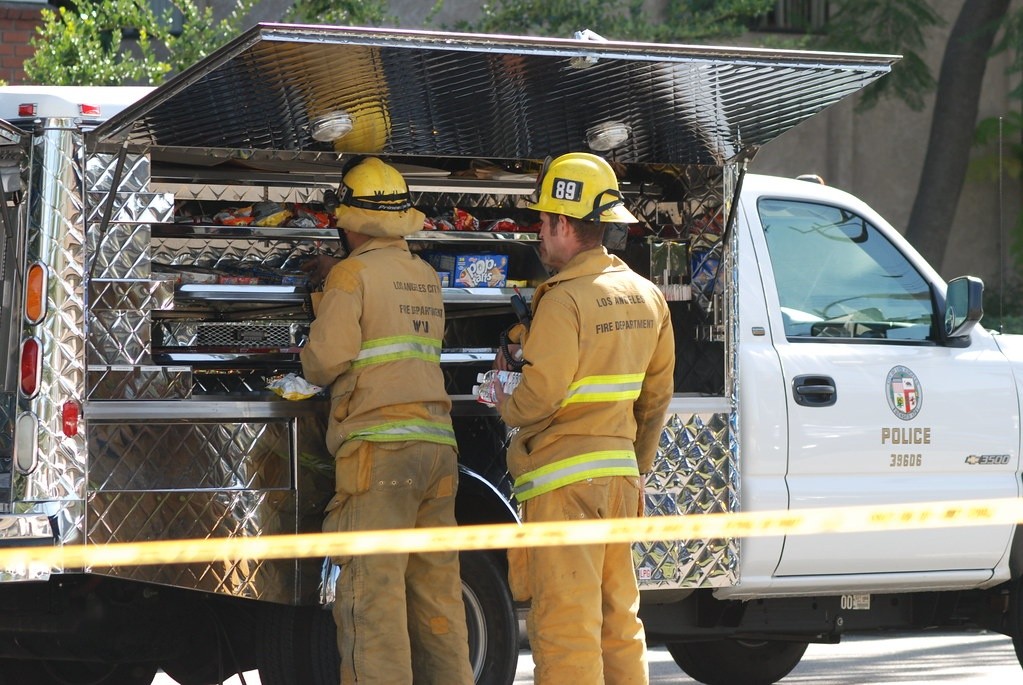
xmin=152 ymin=180 xmax=544 ymax=368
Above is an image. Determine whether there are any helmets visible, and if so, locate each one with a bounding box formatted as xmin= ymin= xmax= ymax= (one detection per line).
xmin=526 ymin=152 xmax=640 ymax=224
xmin=334 ymin=156 xmax=426 ymax=237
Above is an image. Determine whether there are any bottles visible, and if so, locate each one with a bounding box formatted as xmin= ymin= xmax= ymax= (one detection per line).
xmin=473 ymin=382 xmax=517 ymax=404
xmin=476 ymin=370 xmax=522 ymax=386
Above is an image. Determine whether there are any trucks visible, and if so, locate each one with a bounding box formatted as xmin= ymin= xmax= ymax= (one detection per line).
xmin=1 ymin=21 xmax=1023 ymax=685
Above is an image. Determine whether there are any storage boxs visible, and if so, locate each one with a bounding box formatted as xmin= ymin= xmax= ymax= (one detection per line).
xmin=152 ymin=248 xmax=529 ymax=289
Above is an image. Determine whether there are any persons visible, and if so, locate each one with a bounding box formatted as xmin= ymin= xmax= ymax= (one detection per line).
xmin=495 ymin=152 xmax=675 ymax=685
xmin=300 ymin=157 xmax=474 ymax=685
